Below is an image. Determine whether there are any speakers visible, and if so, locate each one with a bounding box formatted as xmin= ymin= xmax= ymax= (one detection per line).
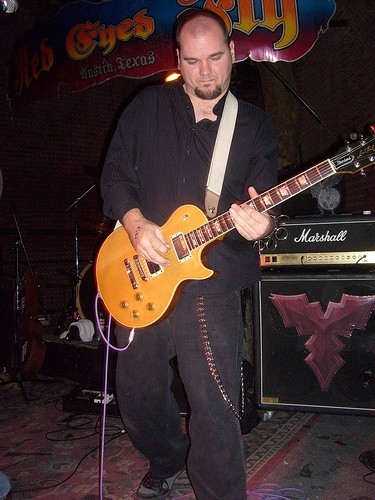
xmin=256 ymin=273 xmax=375 ymax=417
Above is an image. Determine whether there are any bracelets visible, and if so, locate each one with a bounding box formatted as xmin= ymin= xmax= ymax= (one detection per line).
xmin=263 ymin=214 xmax=276 ymax=240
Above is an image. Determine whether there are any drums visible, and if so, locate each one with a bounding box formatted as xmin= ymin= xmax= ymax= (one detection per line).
xmin=76 ymin=262 xmax=116 ymax=331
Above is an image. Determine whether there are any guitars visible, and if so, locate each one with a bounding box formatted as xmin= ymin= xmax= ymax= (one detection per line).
xmin=96 ymin=125 xmax=375 ymax=329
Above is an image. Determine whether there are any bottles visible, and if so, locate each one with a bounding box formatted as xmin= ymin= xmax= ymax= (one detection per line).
xmin=96 ymin=312 xmax=104 ymax=340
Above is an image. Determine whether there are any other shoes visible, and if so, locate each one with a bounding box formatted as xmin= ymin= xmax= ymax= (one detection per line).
xmin=136 ymin=467 xmax=185 ymax=500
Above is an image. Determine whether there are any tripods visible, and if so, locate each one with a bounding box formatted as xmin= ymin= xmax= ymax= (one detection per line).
xmin=0 ymin=203 xmax=56 ymax=404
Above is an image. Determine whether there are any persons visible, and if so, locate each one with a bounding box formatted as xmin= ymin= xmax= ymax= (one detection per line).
xmin=100 ymin=8 xmax=277 ymax=500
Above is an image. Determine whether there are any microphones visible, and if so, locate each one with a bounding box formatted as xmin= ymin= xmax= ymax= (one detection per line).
xmin=0 ymin=0 xmax=18 ymax=14
